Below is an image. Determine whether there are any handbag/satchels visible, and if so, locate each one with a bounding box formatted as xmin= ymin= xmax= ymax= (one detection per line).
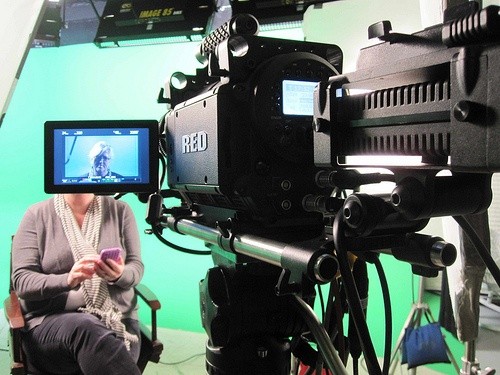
xmin=402 ymin=304 xmax=417 ymax=363
xmin=407 ymin=308 xmax=452 ymax=369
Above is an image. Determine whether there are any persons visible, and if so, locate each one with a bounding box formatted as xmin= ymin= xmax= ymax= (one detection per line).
xmin=84 ymin=140 xmax=121 ymax=177
xmin=11 ymin=192 xmax=145 ymax=375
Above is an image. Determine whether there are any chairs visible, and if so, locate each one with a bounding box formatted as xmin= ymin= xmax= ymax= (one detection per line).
xmin=3 ymin=233 xmax=164 ymax=375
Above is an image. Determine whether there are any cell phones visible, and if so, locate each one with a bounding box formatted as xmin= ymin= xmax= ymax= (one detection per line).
xmin=100 ymin=248 xmax=120 ymax=266
